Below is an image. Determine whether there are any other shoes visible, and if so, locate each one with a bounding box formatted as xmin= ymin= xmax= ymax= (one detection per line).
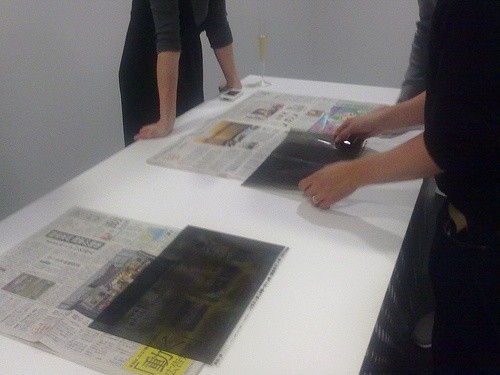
xmin=411 ymin=309 xmax=437 ymax=348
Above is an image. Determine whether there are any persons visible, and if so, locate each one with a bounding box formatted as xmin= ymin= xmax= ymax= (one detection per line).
xmin=119 ymin=1 xmax=242 ymax=149
xmin=298 ymin=0 xmax=500 ymax=374
xmin=383 ymin=0 xmax=446 ymax=349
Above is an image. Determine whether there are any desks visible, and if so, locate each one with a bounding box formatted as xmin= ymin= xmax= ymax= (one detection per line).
xmin=0 ymin=74 xmax=436 ymax=375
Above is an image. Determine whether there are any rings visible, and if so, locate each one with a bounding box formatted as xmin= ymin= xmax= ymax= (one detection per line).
xmin=312 ymin=195 xmax=319 ymax=206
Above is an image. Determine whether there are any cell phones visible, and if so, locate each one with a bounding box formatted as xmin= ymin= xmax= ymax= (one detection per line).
xmin=220 ymin=88 xmax=245 ymax=101
